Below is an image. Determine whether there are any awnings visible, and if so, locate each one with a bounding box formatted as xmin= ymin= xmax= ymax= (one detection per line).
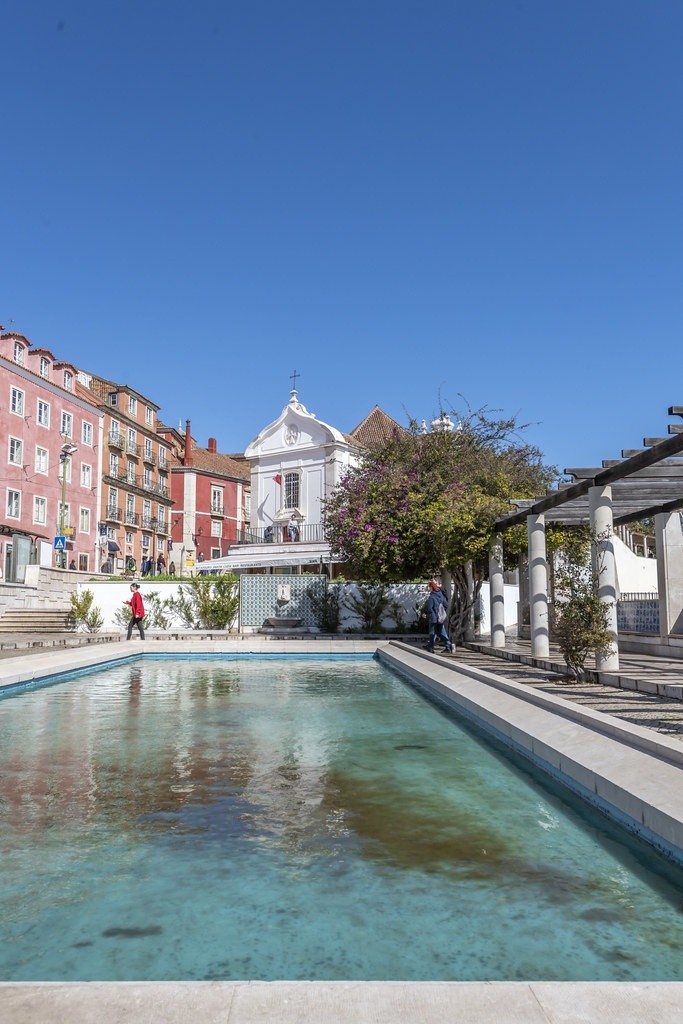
xmin=108 ymin=541 xmax=120 ymax=551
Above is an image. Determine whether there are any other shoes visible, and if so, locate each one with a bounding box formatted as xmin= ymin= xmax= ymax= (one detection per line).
xmin=441 ymin=651 xmax=451 ymax=653
xmin=452 ymin=644 xmax=456 ymax=654
xmin=423 ymin=645 xmax=427 ymax=648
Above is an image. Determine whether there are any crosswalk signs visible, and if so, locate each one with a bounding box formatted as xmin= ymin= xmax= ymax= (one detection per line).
xmin=54 ymin=536 xmax=66 ymax=550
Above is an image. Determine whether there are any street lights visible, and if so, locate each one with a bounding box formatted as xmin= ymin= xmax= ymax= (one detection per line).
xmin=148 ymin=517 xmax=157 ymax=577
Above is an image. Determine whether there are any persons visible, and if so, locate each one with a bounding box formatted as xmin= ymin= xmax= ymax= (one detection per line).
xmin=287 ymin=514 xmax=299 ymax=542
xmin=264 ymin=526 xmax=273 ymax=543
xmin=422 ymin=576 xmax=457 ymax=654
xmin=122 ymin=583 xmax=146 ymax=640
xmin=61 ymin=552 xmax=176 ymax=577
xmin=196 ymin=552 xmax=204 ymax=578
